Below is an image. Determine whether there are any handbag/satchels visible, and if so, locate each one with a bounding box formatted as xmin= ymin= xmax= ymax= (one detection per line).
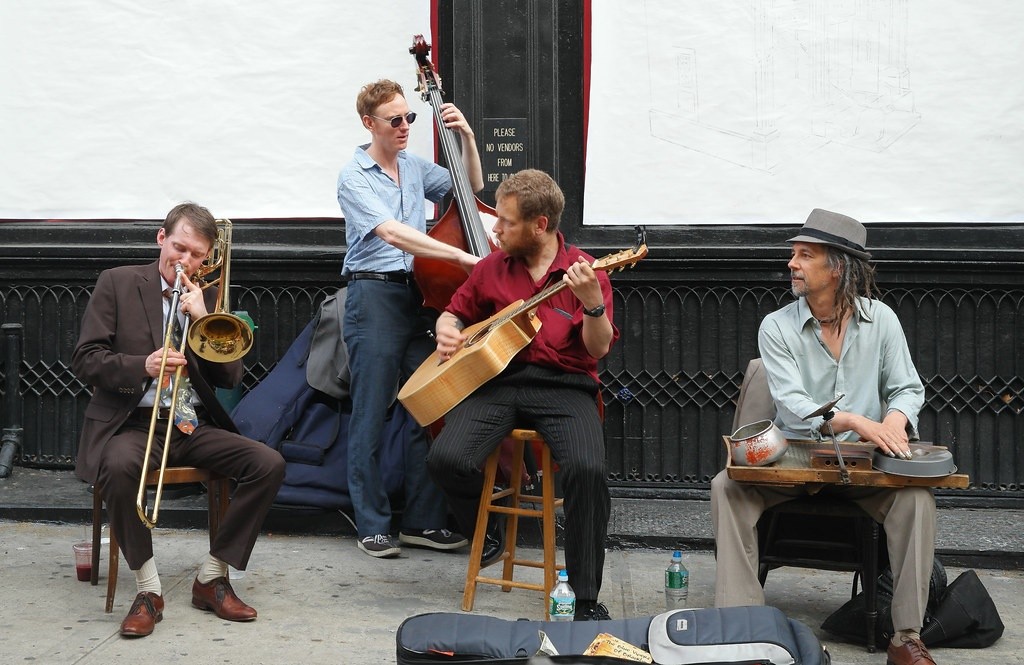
xmin=820 ymin=554 xmax=1004 ymax=649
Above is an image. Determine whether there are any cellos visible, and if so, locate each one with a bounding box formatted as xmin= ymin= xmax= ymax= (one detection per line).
xmin=408 ymin=29 xmax=606 ymax=546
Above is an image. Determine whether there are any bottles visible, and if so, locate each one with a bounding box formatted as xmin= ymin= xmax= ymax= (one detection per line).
xmin=665 ymin=550 xmax=689 ymax=612
xmin=549 ymin=569 xmax=576 ymax=622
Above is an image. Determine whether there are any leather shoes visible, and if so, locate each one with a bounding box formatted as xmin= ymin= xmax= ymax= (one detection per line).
xmin=479 ymin=495 xmax=506 ymax=565
xmin=191 ymin=573 xmax=257 ymax=621
xmin=120 ymin=592 xmax=164 ymax=637
xmin=887 ymin=634 xmax=936 ymax=665
xmin=575 ymin=602 xmax=611 ymax=622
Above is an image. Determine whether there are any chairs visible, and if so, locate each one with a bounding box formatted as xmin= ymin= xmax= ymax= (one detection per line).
xmin=731 ymin=357 xmax=882 ymax=657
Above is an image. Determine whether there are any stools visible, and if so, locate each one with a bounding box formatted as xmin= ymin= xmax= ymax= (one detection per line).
xmin=462 ymin=431 xmax=565 ymax=620
xmin=91 ymin=467 xmax=236 ymax=615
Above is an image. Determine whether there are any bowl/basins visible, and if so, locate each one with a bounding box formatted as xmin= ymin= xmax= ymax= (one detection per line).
xmin=729 ymin=419 xmax=789 ymax=466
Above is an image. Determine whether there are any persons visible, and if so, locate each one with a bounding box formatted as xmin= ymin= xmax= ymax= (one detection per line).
xmin=711 ymin=208 xmax=939 ymax=665
xmin=436 ymin=169 xmax=620 ymax=623
xmin=338 ymin=79 xmax=485 ymax=557
xmin=72 ymin=202 xmax=286 ymax=633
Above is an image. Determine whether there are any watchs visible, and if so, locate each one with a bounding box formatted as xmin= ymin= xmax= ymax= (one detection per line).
xmin=583 ymin=303 xmax=606 ymax=317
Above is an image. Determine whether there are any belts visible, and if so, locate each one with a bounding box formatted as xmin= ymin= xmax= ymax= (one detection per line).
xmin=137 ymin=408 xmax=174 ymax=421
xmin=350 ymin=272 xmax=416 ymax=287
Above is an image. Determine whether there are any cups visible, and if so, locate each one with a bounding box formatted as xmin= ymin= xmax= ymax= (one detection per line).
xmin=73 ymin=543 xmax=93 ymax=582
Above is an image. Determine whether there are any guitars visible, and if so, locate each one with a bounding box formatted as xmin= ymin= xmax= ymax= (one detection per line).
xmin=396 ymin=223 xmax=651 ymax=429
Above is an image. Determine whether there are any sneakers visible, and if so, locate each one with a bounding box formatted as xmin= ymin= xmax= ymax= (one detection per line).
xmin=358 ymin=534 xmax=402 ymax=558
xmin=399 ymin=524 xmax=469 ymax=551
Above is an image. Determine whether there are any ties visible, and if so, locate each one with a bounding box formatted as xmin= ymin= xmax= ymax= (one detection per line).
xmin=166 ymin=296 xmax=241 ymax=435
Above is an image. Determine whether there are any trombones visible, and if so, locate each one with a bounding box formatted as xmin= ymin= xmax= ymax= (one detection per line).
xmin=132 ymin=216 xmax=256 ymax=530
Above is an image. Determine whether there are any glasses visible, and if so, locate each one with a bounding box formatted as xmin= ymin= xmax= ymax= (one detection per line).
xmin=371 ymin=111 xmax=417 ymax=128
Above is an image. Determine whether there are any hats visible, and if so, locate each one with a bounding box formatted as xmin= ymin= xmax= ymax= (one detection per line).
xmin=786 ymin=208 xmax=872 ymax=262
